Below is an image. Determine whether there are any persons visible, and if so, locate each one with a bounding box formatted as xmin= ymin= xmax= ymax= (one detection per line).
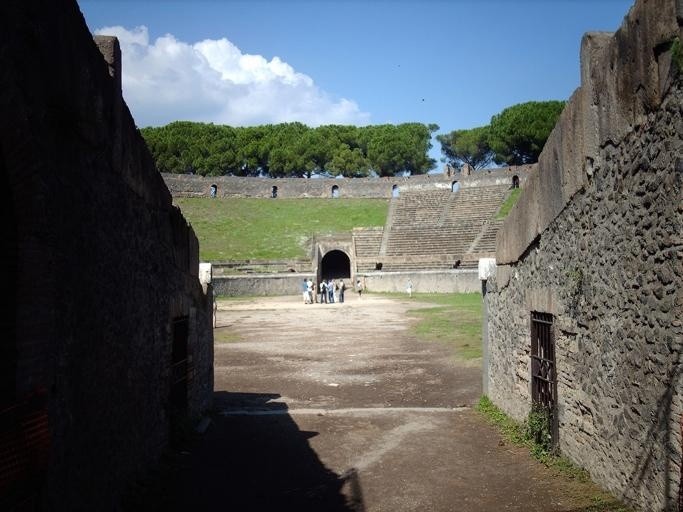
xmin=406 ymin=278 xmax=413 ymax=298
xmin=303 ymin=278 xmax=345 ymax=304
xmin=357 ymin=281 xmax=362 ymax=298
